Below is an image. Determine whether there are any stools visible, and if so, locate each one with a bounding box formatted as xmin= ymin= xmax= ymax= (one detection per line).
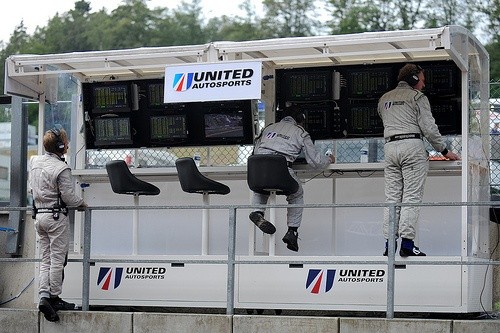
xmin=106 ymin=160 xmax=160 ymax=257
xmin=176 ymin=157 xmax=231 ymax=254
xmin=247 ymin=154 xmax=299 ymax=256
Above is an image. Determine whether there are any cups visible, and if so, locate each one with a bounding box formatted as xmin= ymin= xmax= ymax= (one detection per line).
xmin=368 ymin=138 xmax=377 ymax=163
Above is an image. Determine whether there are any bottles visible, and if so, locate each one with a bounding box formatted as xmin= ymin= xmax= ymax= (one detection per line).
xmin=193 ymin=152 xmax=201 ymax=166
xmin=325 ymin=148 xmax=334 ymax=156
xmin=360 ymin=146 xmax=368 ymax=162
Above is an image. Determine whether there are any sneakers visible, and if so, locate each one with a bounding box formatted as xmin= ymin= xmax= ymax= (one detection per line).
xmin=249 ymin=211 xmax=276 ymax=234
xmin=282 ymin=229 xmax=301 ymax=252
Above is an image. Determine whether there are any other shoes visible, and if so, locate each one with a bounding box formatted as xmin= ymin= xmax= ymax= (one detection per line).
xmin=39 ymin=297 xmax=75 ymax=322
xmin=383 ymin=249 xmax=396 ymax=256
xmin=400 ymin=246 xmax=427 ymax=257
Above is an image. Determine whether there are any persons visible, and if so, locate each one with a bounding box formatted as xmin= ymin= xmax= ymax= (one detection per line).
xmin=248 ymin=108 xmax=335 ymax=251
xmin=377 ymin=64 xmax=460 ymax=257
xmin=30 ymin=128 xmax=88 ymax=322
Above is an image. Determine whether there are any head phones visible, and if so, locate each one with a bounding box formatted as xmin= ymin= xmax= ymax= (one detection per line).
xmin=50 ymin=127 xmax=65 ymax=153
xmin=300 ymin=110 xmax=309 ymax=121
xmin=409 ymin=65 xmax=422 ymax=85
